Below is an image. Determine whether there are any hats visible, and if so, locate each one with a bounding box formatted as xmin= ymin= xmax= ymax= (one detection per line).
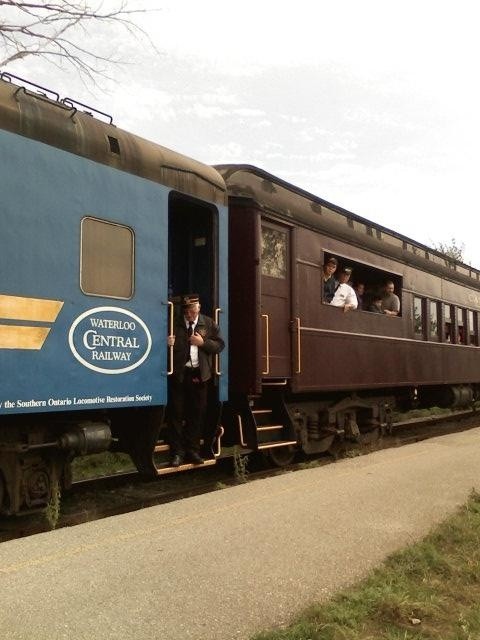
xmin=181 ymin=294 xmax=199 ymax=306
xmin=327 ymin=257 xmax=337 ymax=264
xmin=340 ymin=266 xmax=352 ymax=274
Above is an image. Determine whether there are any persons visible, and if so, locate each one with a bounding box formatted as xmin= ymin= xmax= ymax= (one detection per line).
xmin=166 ymin=294 xmax=225 ymax=467
xmin=445 ymin=324 xmax=451 ymax=342
xmin=369 ymin=297 xmax=386 ymax=314
xmin=324 ymin=257 xmax=339 ymax=306
xmin=353 ymin=282 xmax=365 ymax=311
xmin=377 ymin=281 xmax=401 ymax=316
xmin=330 ymin=266 xmax=359 ymax=311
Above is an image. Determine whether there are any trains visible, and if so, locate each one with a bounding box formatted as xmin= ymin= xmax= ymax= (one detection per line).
xmin=0 ymin=70 xmax=480 ymax=522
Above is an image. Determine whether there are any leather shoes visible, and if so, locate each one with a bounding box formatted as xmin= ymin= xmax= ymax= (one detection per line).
xmin=170 ymin=455 xmax=182 ymax=467
xmin=185 ymin=449 xmax=200 ymax=463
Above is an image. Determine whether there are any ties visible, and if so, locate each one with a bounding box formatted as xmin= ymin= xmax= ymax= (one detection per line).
xmin=334 ymin=284 xmax=339 ymax=292
xmin=188 ymin=321 xmax=194 ymax=336
